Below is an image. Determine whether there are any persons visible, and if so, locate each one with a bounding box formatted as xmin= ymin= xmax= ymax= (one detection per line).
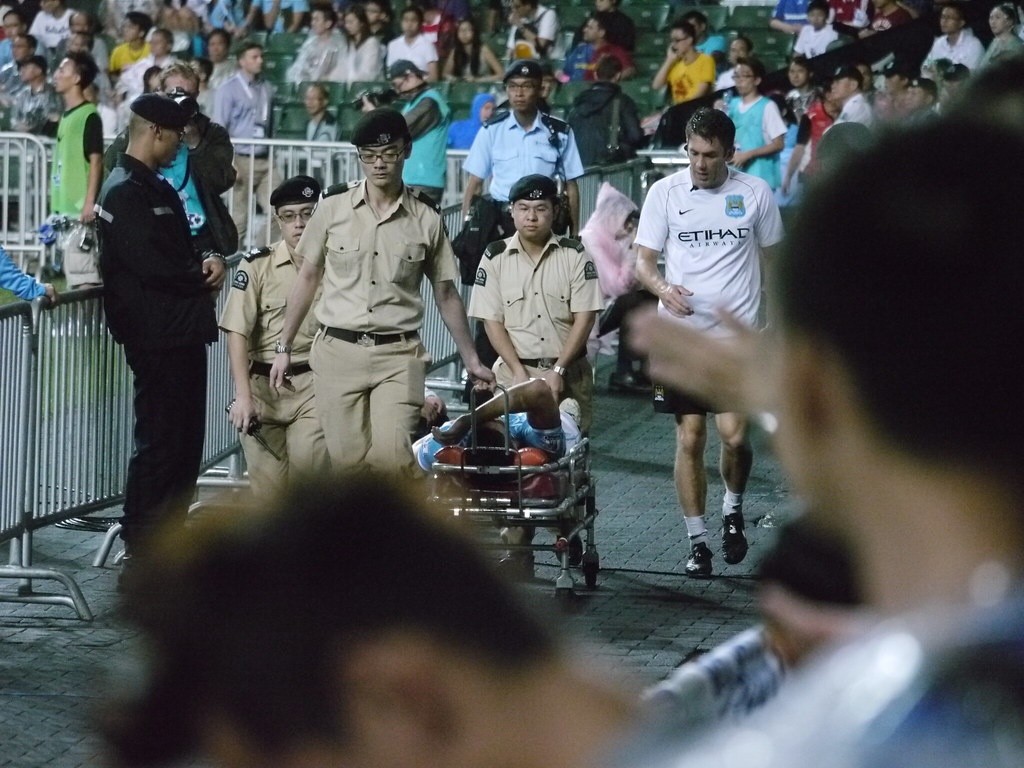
xmin=634 ymin=107 xmax=787 ymax=578
xmin=92 ymin=93 xmax=227 ymax=595
xmin=101 ymin=62 xmax=239 ymax=259
xmin=270 ymin=107 xmax=497 ymax=486
xmin=468 ymin=173 xmax=606 ymax=579
xmin=218 ymin=175 xmax=325 ymax=500
xmin=0 ymin=0 xmax=1024 ymax=768
xmin=50 ymin=50 xmax=104 ymax=337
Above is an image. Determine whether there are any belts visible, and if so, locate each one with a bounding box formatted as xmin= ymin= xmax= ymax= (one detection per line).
xmin=519 ymin=357 xmax=558 ymax=369
xmin=250 ymin=360 xmax=311 ymax=377
xmin=320 ymin=323 xmax=418 ymax=347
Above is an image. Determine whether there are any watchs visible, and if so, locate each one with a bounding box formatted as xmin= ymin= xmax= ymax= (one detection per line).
xmin=275 ymin=340 xmax=291 ymax=353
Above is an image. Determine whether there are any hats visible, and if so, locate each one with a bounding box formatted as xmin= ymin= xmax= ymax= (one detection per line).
xmin=350 ymin=109 xmax=406 ymax=145
xmin=131 ymin=93 xmax=189 ymax=128
xmin=830 ymin=64 xmax=864 ymax=83
xmin=885 ymin=61 xmax=918 ymax=77
xmin=386 ymin=60 xmax=429 ymax=81
xmin=509 ymin=174 xmax=557 ymax=204
xmin=503 ymin=60 xmax=543 ymax=82
xmin=270 ymin=176 xmax=320 ymax=208
xmin=943 ymin=64 xmax=969 ymax=79
xmin=904 ymin=77 xmax=937 ymax=96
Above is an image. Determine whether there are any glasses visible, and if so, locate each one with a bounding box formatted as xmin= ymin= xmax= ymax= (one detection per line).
xmin=670 ymin=35 xmax=689 ymax=43
xmin=274 ymin=208 xmax=315 ymax=222
xmin=357 ymin=145 xmax=407 ymax=163
xmin=171 ymin=128 xmax=186 ymax=142
xmin=732 ymin=74 xmax=755 ymax=81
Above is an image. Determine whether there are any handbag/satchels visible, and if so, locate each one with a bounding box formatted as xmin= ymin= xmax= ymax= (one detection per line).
xmin=604 ymin=98 xmax=630 ymax=163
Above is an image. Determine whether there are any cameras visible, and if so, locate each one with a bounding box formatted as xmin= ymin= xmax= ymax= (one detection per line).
xmin=166 ymin=86 xmax=199 ymax=120
xmin=80 ymin=233 xmax=93 ymax=251
xmin=352 ymin=89 xmax=381 ymax=110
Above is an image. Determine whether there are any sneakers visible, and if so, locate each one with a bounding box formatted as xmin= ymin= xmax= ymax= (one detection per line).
xmin=722 ymin=512 xmax=748 ymax=565
xmin=558 ymin=396 xmax=580 ymax=429
xmin=685 ymin=542 xmax=713 ymax=578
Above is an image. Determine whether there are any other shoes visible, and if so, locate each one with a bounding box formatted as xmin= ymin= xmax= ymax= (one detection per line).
xmin=498 ymin=552 xmax=535 ymax=579
xmin=116 ymin=541 xmax=135 ymax=596
xmin=555 ymin=533 xmax=582 ymax=566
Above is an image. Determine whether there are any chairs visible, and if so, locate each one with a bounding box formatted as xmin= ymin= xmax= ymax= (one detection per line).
xmin=208 ymin=0 xmax=798 ymax=190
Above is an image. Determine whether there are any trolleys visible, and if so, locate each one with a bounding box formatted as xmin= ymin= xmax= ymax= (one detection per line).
xmin=425 ymin=381 xmax=599 ymax=613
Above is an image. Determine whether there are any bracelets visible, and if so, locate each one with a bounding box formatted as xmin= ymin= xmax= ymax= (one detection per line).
xmin=210 ymin=253 xmax=226 ymax=263
xmin=552 ymin=366 xmax=566 ymax=376
